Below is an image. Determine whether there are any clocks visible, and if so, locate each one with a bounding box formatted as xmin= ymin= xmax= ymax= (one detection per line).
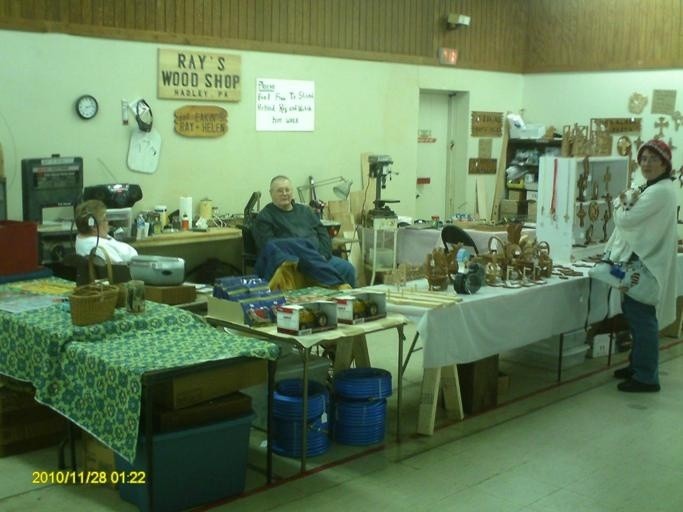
xmin=73 ymin=94 xmax=99 ymax=120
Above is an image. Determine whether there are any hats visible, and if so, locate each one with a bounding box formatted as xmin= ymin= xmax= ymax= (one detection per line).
xmin=636 ymin=139 xmax=672 ymax=175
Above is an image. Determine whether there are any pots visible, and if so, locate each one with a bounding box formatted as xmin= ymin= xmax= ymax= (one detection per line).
xmin=125 ymin=254 xmax=186 ymax=287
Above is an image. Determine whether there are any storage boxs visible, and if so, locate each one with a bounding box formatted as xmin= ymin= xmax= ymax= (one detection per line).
xmin=494 ymin=166 xmax=537 ymax=225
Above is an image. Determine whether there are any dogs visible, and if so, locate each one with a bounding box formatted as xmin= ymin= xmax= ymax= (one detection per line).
xmin=614 ymin=189 xmax=640 ymax=211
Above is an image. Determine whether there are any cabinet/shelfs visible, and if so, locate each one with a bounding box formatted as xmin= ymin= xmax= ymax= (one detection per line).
xmin=533 ymin=152 xmax=636 ymax=266
xmin=491 ymin=111 xmax=589 ymax=226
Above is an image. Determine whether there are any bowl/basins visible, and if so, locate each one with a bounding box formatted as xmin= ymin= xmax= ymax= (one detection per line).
xmin=319 ymin=223 xmax=342 ymax=239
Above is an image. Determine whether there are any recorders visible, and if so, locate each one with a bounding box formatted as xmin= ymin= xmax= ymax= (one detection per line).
xmin=84 ymin=183 xmax=143 ymax=209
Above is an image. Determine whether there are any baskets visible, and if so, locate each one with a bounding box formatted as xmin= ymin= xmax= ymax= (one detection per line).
xmin=68 ymin=279 xmax=120 ymax=327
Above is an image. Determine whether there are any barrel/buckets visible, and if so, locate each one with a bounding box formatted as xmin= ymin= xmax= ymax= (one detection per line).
xmin=369 ymin=247 xmax=394 ymax=268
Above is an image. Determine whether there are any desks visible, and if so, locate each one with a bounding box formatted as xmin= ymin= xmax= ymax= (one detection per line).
xmin=0 ymin=274 xmax=409 ymax=512
xmin=353 ymin=217 xmax=682 ymax=437
xmin=130 ymin=228 xmax=245 ymax=273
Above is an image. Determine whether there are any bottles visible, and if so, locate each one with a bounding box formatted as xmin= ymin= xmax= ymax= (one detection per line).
xmin=126 ymin=277 xmax=146 ymax=315
xmin=182 ymin=212 xmax=189 ymax=231
xmin=200 ymin=197 xmax=213 ymax=220
xmin=136 ymin=214 xmax=145 ymax=228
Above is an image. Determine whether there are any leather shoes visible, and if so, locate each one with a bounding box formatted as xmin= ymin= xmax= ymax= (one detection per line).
xmin=613 ymin=365 xmax=636 ymax=378
xmin=617 ymin=378 xmax=660 ymax=393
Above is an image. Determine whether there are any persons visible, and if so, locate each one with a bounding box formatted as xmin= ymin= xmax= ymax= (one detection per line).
xmin=251 ymin=174 xmax=356 ymax=291
xmin=70 ymin=199 xmax=144 ymax=267
xmin=595 ymin=138 xmax=683 ymax=395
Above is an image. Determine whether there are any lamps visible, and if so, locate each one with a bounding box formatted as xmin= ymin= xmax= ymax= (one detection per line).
xmin=295 ymin=174 xmax=354 ymax=209
xmin=119 ymin=97 xmax=153 ymax=134
xmin=445 ymin=12 xmax=470 ymax=31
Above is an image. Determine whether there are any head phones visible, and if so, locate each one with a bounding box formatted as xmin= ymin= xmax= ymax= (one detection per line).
xmin=76 ymin=199 xmax=94 ymax=235
